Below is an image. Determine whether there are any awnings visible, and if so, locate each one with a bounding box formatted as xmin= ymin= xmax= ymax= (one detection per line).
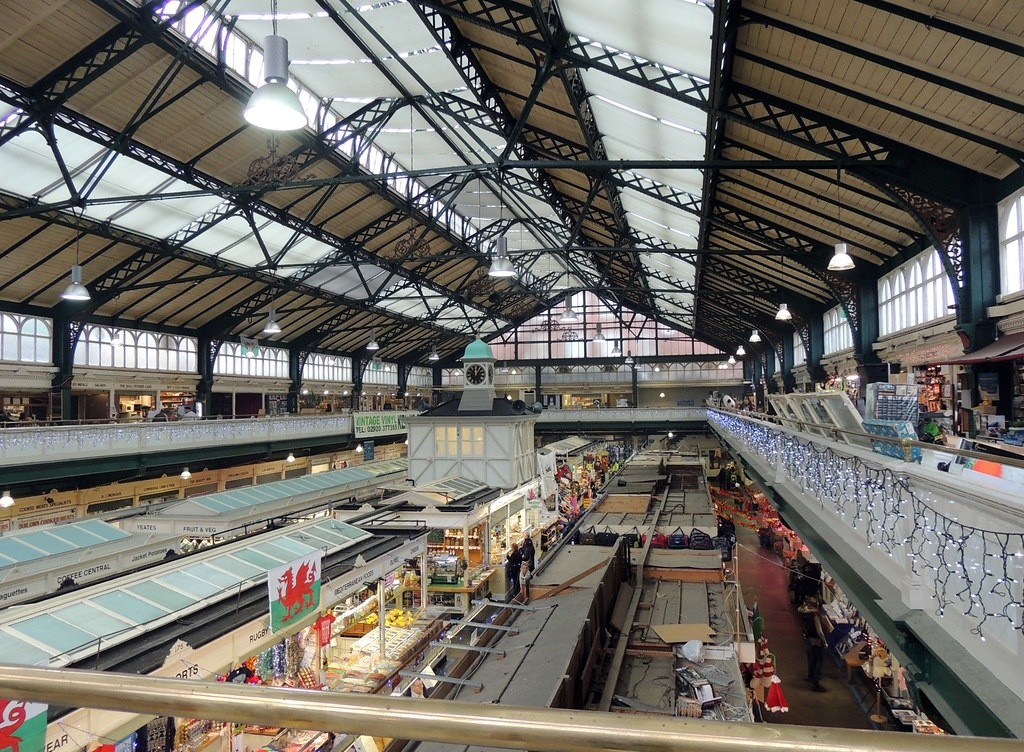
xmin=928 ymin=333 xmax=1024 ymax=367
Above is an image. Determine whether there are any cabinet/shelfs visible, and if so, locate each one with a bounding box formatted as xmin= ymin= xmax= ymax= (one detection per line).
xmin=427 ymin=524 xmax=482 ymax=568
xmin=160 ymin=395 xmax=194 ymax=409
xmin=339 ymin=623 xmax=378 ymax=639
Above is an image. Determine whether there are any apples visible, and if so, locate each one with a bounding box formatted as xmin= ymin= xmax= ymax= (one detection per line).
xmin=299 ymin=668 xmax=316 ymax=688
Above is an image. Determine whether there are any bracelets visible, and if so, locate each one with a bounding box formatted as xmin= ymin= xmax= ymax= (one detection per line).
xmin=824 ymin=641 xmax=827 ymax=644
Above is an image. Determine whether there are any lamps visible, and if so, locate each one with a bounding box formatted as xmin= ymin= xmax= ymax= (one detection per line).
xmin=560 ymin=250 xmax=634 ymax=364
xmin=718 ymin=166 xmax=857 ymax=370
xmin=366 ymin=306 xmax=380 ymax=350
xmin=0 ymin=491 xmax=16 ymax=508
xmin=286 ymin=452 xmax=295 ymax=463
xmin=241 ymin=0 xmax=308 ymax=131
xmin=428 ymin=326 xmax=440 ymax=360
xmin=179 ymin=467 xmax=192 ymax=481
xmin=263 ymin=287 xmax=281 ymax=333
xmin=356 ymin=444 xmax=363 ymax=452
xmin=60 ymin=222 xmax=92 ymax=301
xmin=490 ymin=166 xmax=518 ymax=277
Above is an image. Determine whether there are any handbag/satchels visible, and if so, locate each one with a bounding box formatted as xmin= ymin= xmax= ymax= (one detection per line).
xmin=621 ymin=525 xmax=731 ymax=550
xmin=568 ymin=526 xmax=619 ymax=546
xmin=718 ymin=514 xmax=735 ymax=548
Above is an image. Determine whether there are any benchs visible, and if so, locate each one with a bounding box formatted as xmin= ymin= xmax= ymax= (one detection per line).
xmin=978 ymin=434 xmax=1004 ymax=443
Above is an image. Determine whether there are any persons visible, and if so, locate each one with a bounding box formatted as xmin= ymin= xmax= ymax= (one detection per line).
xmin=506 ymin=535 xmax=535 ymax=597
xmin=571 ymin=444 xmax=628 ymax=516
xmin=712 ymin=452 xmax=829 ymax=692
xmin=0 ymin=411 xmax=34 ymax=428
xmin=706 ymin=394 xmax=755 ymax=417
xmin=153 ymin=407 xmax=198 ymax=422
xmin=325 ymin=400 xmax=393 ymax=412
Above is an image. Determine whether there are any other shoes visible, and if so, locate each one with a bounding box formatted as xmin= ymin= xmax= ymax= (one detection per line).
xmin=813 ymin=684 xmax=830 ymax=692
xmin=803 ymin=677 xmax=814 ymax=682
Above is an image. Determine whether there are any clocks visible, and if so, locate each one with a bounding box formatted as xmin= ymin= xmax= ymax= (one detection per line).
xmin=459 ymin=327 xmax=500 ymax=389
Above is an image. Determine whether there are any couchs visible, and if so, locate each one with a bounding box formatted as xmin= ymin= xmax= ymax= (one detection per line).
xmin=677 ymin=400 xmax=694 ymax=407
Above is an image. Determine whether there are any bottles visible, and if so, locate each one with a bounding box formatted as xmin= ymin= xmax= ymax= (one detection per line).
xmin=429 ymin=528 xmax=478 ymax=555
